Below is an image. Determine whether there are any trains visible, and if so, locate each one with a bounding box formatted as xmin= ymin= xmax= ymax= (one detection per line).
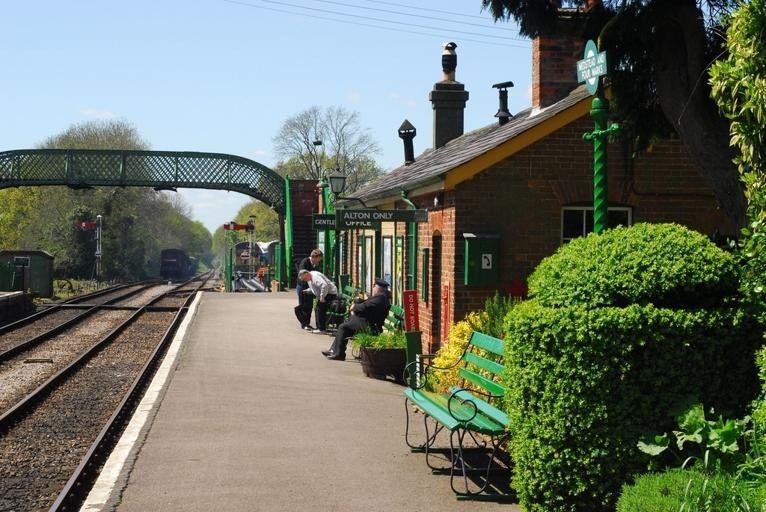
xmin=160 ymin=249 xmax=199 ymax=279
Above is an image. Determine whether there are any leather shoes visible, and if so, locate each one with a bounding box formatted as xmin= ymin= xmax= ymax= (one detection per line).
xmin=321 ymin=349 xmax=334 ymax=357
xmin=327 ymin=354 xmax=346 ymax=361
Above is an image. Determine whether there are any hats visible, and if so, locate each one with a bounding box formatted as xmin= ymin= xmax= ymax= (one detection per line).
xmin=374 ymin=277 xmax=390 ymax=291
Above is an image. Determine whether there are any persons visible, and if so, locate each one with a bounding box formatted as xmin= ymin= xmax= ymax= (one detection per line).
xmin=298 ymin=270 xmax=337 ymax=334
xmin=297 ymin=249 xmax=323 ymax=331
xmin=322 ymin=277 xmax=390 ymax=360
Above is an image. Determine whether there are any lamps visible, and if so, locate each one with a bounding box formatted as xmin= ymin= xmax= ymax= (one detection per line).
xmin=330 ymin=161 xmax=368 ymax=208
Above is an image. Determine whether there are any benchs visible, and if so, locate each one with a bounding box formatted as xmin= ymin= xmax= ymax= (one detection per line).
xmin=402 ymin=330 xmax=517 ymax=502
xmin=324 ymin=285 xmax=404 ymax=362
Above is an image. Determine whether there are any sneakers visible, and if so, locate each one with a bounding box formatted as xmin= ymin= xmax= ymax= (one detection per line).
xmin=312 ymin=329 xmax=326 ymax=335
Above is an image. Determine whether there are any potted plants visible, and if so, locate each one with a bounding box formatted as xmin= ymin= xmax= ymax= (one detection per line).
xmin=348 ymin=323 xmax=405 ymax=379
